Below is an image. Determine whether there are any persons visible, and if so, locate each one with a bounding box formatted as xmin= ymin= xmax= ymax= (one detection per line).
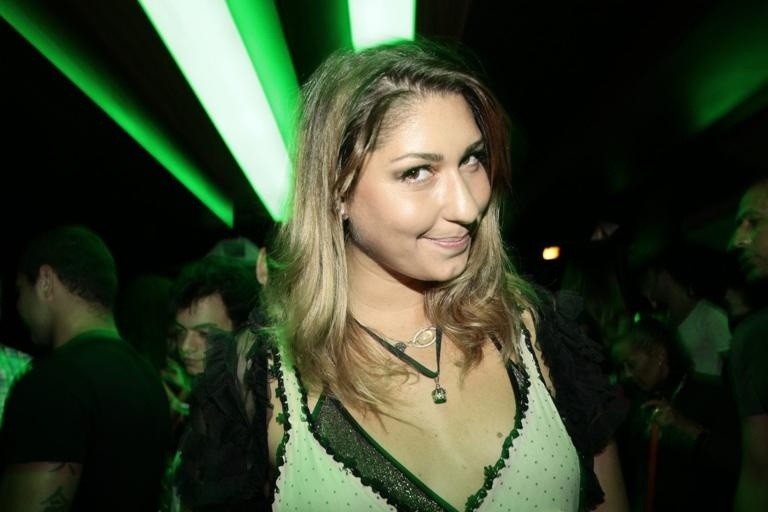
xmin=0 ymin=182 xmax=768 ymax=510
xmin=171 ymin=35 xmax=628 ymax=511
